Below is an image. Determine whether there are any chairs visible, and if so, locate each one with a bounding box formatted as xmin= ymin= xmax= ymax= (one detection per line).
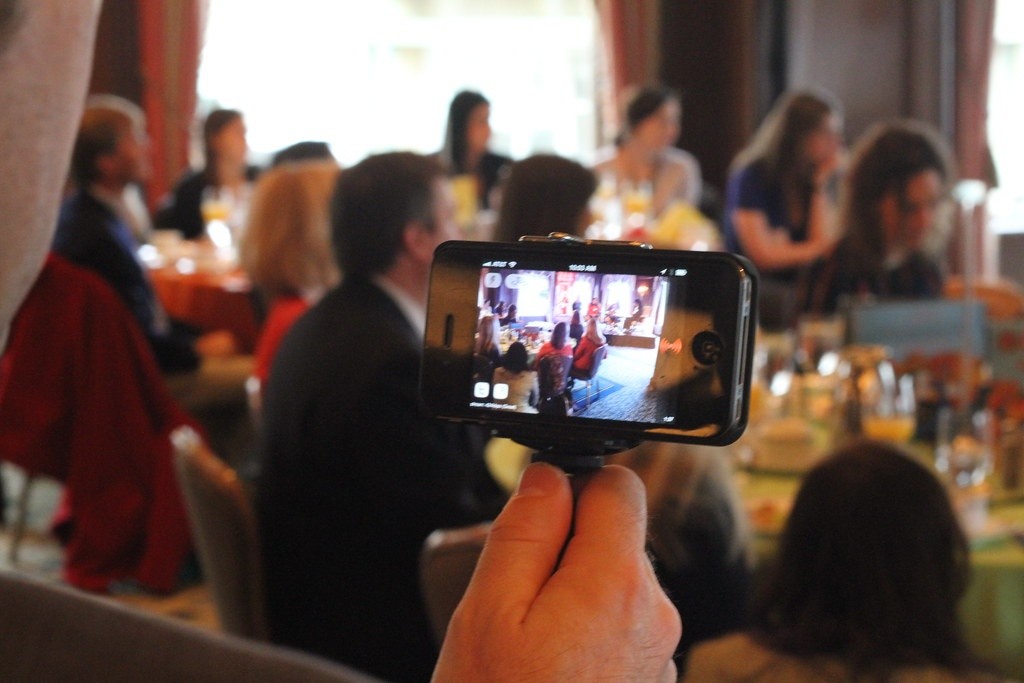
xmin=538 ymin=355 xmax=571 ymax=400
xmin=570 ymin=343 xmax=609 ymax=409
xmin=123 ymin=182 xmax=153 ymax=236
xmin=170 ymin=429 xmax=266 ymax=641
xmin=628 ymin=317 xmax=645 ymax=336
xmin=472 ymin=354 xmax=494 ymax=386
xmin=418 ymin=522 xmax=500 ymax=649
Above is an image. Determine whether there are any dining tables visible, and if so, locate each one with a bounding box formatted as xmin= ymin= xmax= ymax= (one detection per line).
xmin=475 ymin=328 xmax=577 ymax=368
xmin=725 ymin=371 xmax=1024 ymax=681
xmin=146 ymin=239 xmax=256 ymax=346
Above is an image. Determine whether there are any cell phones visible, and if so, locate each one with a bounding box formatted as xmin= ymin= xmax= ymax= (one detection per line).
xmin=421 ymin=238 xmax=760 ymax=447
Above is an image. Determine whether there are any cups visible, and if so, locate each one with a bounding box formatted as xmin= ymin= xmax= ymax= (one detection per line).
xmin=752 ymin=348 xmax=796 ymax=432
xmin=799 ymin=352 xmax=853 ymax=433
xmin=622 ymin=180 xmax=653 ymax=216
xmin=860 ymin=362 xmax=917 ymax=441
xmin=937 ymin=408 xmax=996 ymax=492
xmin=202 ymin=187 xmax=235 ymax=222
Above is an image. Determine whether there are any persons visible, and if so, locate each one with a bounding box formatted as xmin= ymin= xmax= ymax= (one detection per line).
xmin=0 ymin=0 xmax=1009 ymax=683
xmin=624 ymin=299 xmax=643 ymax=332
xmin=500 ymin=304 xmax=517 ymax=327
xmin=586 ymin=298 xmax=602 ymax=318
xmin=493 ymin=342 xmax=540 ymax=407
xmin=492 ymin=301 xmax=504 ymax=313
xmin=532 ymin=322 xmax=573 ymax=371
xmin=473 ymin=316 xmax=500 ymax=361
xmin=567 ymin=319 xmax=606 ymax=388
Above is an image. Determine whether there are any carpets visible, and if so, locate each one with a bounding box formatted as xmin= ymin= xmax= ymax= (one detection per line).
xmin=571 ymin=374 xmax=623 ymax=409
